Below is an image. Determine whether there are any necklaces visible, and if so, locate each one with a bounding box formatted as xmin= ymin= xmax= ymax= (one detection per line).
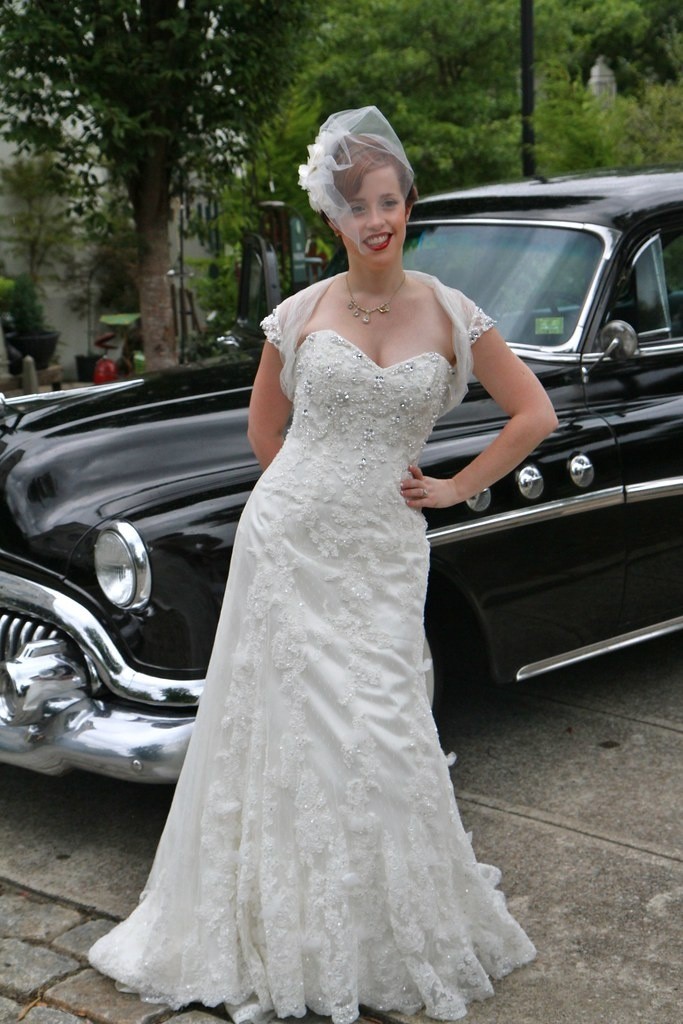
xmin=345 ymin=271 xmax=406 ymax=324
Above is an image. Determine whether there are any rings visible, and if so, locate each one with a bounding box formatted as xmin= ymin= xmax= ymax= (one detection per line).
xmin=421 ymin=488 xmax=429 ymax=497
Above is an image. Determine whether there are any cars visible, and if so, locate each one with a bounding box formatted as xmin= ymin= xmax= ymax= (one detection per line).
xmin=1 ymin=161 xmax=683 ymax=797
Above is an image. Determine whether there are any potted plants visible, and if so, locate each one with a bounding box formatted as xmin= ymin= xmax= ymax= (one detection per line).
xmin=53 ymin=248 xmax=114 ymax=383
xmin=4 ymin=270 xmax=61 ymax=375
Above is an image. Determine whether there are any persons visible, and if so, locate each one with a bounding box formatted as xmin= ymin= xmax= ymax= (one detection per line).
xmin=86 ymin=101 xmax=562 ymax=1024
